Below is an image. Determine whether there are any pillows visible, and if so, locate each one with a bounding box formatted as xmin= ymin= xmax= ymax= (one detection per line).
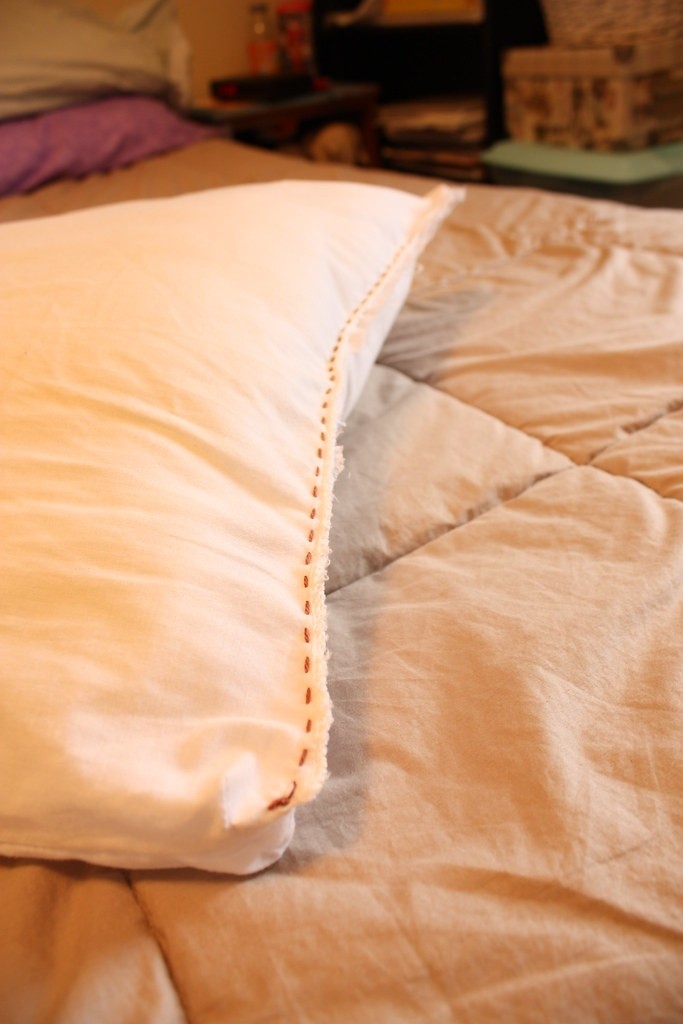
xmin=0 ymin=134 xmax=465 ymax=880
xmin=0 ymin=96 xmax=195 ymax=195
xmin=0 ymin=0 xmax=189 ymax=122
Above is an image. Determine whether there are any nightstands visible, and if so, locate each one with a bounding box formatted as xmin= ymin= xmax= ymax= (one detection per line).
xmin=185 ymin=83 xmax=377 ymax=166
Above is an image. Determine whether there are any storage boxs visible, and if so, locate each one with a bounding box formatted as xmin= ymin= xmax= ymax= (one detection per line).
xmin=376 ymin=96 xmax=488 ymax=150
xmin=500 ymin=39 xmax=683 ymax=152
xmin=477 ymin=140 xmax=683 ymax=209
xmin=383 ymin=147 xmax=491 ymax=185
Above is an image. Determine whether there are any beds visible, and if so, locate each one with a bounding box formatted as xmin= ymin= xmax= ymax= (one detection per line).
xmin=0 ymin=134 xmax=683 ymax=1024
